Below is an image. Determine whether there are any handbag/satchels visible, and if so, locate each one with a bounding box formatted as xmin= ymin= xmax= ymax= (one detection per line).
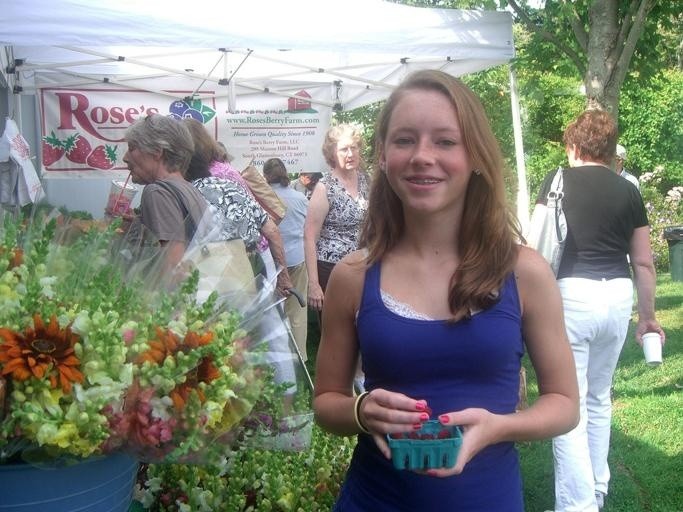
xmin=521 ymin=203 xmax=568 ymax=280
xmin=125 ymin=237 xmax=258 ymax=322
xmin=239 ymin=164 xmax=287 ymax=229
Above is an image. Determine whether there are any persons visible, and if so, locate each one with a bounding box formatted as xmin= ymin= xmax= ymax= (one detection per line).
xmin=610 ymin=141 xmax=641 ymax=325
xmin=533 ymin=104 xmax=668 ymax=511
xmin=291 ymin=173 xmax=311 ymax=196
xmin=307 ymin=69 xmax=583 ymax=510
xmin=177 ymin=118 xmax=293 ymax=303
xmin=104 ymin=116 xmax=222 ymax=294
xmin=206 ymin=142 xmax=298 ymax=421
xmin=264 ymin=158 xmax=310 ymax=368
xmin=302 ymin=124 xmax=373 ymax=398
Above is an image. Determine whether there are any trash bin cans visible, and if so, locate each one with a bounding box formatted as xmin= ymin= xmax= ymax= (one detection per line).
xmin=664 ymin=226 xmax=683 ymax=282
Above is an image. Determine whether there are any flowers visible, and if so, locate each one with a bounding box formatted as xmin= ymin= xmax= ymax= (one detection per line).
xmin=0 ymin=202 xmax=354 ymax=510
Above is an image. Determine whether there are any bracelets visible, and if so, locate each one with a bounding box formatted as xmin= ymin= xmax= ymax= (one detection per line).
xmin=353 ymin=391 xmax=373 ymax=436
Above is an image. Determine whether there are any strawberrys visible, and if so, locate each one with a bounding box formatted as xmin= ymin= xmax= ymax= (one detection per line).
xmin=63 ymin=133 xmax=92 ymax=164
xmin=41 ymin=131 xmax=66 ymax=166
xmin=87 ymin=143 xmax=118 ymax=170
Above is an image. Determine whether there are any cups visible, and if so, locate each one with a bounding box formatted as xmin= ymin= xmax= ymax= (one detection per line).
xmin=641 ymin=332 xmax=663 ymax=366
xmin=104 ymin=180 xmax=138 ymax=223
